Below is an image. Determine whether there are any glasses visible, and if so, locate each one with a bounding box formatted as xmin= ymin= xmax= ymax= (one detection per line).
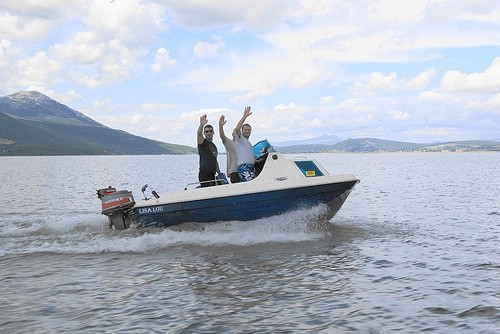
xmin=204 ymin=130 xmax=213 ymax=133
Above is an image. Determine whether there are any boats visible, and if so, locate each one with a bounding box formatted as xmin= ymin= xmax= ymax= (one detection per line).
xmin=96 ymin=139 xmax=361 ymax=232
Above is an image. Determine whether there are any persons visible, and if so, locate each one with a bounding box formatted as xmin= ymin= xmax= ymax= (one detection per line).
xmin=198 ymin=115 xmax=224 ymax=188
xmin=219 ymin=113 xmax=241 ymax=183
xmin=232 ymin=106 xmax=264 ymax=182
